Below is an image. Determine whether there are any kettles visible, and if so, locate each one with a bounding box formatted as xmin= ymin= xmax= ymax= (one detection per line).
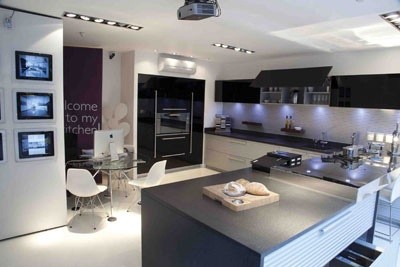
xmin=290 ymin=89 xmax=300 ymax=103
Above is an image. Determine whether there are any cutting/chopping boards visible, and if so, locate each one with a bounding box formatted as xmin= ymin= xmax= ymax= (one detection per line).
xmin=202 ymin=179 xmax=280 ymax=211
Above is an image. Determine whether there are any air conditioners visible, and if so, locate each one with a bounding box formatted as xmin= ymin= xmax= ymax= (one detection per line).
xmin=157 ymin=52 xmax=197 ymax=75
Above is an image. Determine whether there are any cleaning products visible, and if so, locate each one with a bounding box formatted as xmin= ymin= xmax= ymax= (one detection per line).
xmin=285 ymin=115 xmax=289 ymax=128
xmin=289 ymin=115 xmax=293 ymax=129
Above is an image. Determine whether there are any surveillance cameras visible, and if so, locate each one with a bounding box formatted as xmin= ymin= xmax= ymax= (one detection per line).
xmin=109 ymin=52 xmax=115 ymax=59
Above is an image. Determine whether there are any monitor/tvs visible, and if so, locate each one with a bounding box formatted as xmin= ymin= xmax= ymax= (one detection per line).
xmin=15 ymin=50 xmax=52 ymax=81
xmin=157 ymin=113 xmax=191 ymax=139
xmin=18 ymin=131 xmax=54 ymax=159
xmin=16 ymin=92 xmax=52 ymax=119
xmin=93 ymin=129 xmax=125 ymax=163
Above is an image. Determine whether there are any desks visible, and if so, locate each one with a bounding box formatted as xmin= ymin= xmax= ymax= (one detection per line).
xmin=67 ymin=159 xmax=139 ymax=221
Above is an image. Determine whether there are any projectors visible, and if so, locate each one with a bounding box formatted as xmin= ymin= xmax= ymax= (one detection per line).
xmin=176 ymin=0 xmax=221 ymax=20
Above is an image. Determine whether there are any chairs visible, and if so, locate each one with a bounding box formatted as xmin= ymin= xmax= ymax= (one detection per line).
xmin=127 ymin=159 xmax=167 ymax=212
xmin=67 ymin=168 xmax=109 ymax=230
xmin=373 ymin=175 xmax=400 ymax=242
xmin=117 ymin=144 xmax=134 ymax=197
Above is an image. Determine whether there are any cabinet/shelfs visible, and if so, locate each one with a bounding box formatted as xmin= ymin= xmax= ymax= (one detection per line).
xmin=249 ymin=66 xmax=332 ymax=105
xmin=204 ymin=133 xmax=321 ymax=172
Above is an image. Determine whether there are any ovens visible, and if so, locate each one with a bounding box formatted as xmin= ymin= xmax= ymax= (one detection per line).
xmin=156 ymin=112 xmax=191 ymax=157
